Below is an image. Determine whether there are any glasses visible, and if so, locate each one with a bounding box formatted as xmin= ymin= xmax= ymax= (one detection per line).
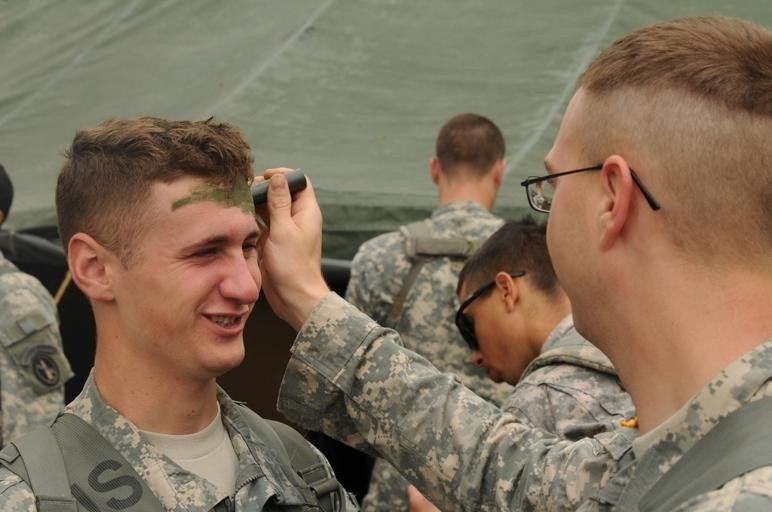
xmin=517 ymin=157 xmax=661 ymax=215
xmin=451 ymin=269 xmax=528 ymax=351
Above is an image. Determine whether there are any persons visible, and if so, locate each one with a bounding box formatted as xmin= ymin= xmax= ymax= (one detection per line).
xmin=400 ymin=209 xmax=637 ymax=512
xmin=2 ymin=115 xmax=364 ymax=511
xmin=243 ymin=16 xmax=772 ymax=512
xmin=343 ymin=111 xmax=515 ymax=511
xmin=2 ymin=169 xmax=75 ymax=410
xmin=530 ymin=177 xmax=548 ymax=208
